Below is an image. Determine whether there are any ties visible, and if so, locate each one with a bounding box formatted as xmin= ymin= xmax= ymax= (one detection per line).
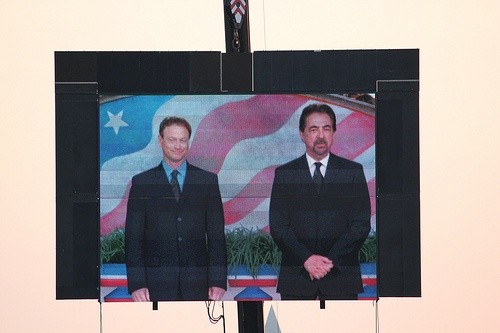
xmin=170 ymin=169 xmax=181 ymax=203
xmin=313 ymin=162 xmax=324 ymax=191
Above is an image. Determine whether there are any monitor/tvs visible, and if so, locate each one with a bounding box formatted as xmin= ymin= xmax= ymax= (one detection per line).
xmin=99 ymin=91 xmax=379 ymax=303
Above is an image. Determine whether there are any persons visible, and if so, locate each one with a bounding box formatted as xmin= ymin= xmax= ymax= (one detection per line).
xmin=266 ymin=102 xmax=373 ymax=301
xmin=123 ymin=114 xmax=229 ymax=301
